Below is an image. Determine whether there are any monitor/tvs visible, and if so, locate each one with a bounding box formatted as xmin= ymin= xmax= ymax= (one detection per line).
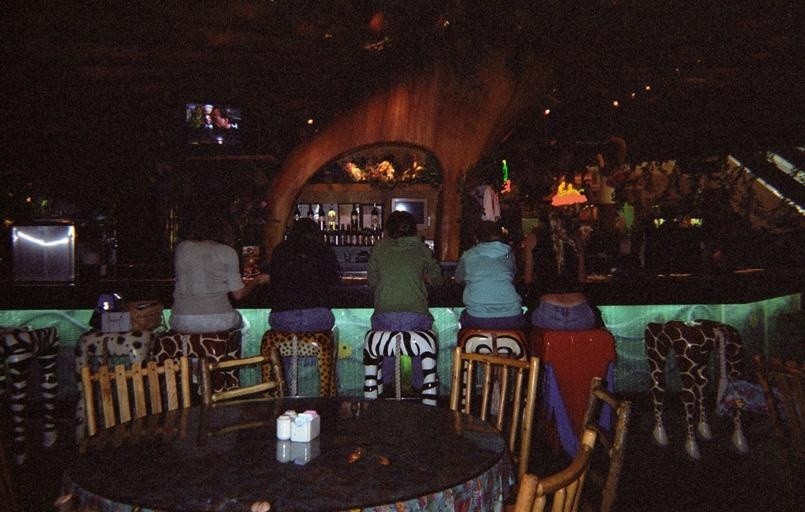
xmin=183 ymin=96 xmax=258 ymax=144
xmin=392 ymin=198 xmax=428 ymax=230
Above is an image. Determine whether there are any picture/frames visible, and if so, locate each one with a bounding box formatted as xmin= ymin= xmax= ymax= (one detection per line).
xmin=295 ymin=200 xmax=385 ymax=246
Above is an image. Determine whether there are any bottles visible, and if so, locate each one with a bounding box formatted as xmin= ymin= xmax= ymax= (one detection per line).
xmin=291 ymin=200 xmax=381 ymax=246
xmin=276 ymin=440 xmax=321 ymax=466
xmin=276 ymin=410 xmax=321 ymax=443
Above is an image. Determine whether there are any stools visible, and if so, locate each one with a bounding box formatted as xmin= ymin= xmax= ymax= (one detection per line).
xmin=642 ymin=315 xmax=755 ymax=461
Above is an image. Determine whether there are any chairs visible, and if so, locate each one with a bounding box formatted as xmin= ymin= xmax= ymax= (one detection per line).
xmin=566 ymin=372 xmax=638 ymax=512
xmin=751 ymin=351 xmax=804 ymax=512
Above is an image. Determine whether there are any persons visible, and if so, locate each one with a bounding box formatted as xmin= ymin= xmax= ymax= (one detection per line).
xmin=169 ymin=209 xmax=261 ymax=335
xmin=453 ymin=220 xmax=528 ymax=332
xmin=269 ymin=216 xmax=343 ymax=332
xmin=210 ymin=106 xmax=238 ymax=129
xmin=365 ymin=210 xmax=447 ymax=399
xmin=526 ymin=227 xmax=598 ymax=332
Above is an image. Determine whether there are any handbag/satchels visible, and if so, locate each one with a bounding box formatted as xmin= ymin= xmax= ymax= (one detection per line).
xmin=89 ymin=293 xmax=164 ymax=333
